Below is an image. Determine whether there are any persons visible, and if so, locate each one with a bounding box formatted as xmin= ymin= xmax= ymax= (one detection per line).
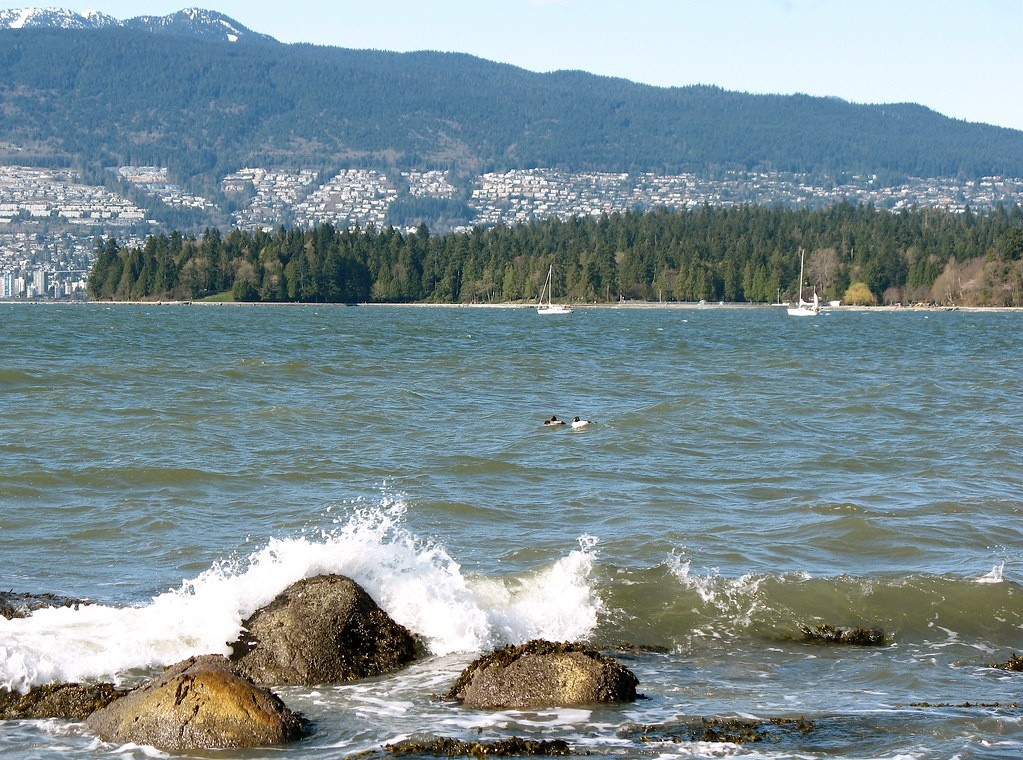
xmin=542 ymin=416 xmax=591 ymax=428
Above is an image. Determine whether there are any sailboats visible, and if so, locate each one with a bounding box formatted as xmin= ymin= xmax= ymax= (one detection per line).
xmin=788 ymin=249 xmax=823 ymax=317
xmin=536 ymin=263 xmax=574 ymax=315
